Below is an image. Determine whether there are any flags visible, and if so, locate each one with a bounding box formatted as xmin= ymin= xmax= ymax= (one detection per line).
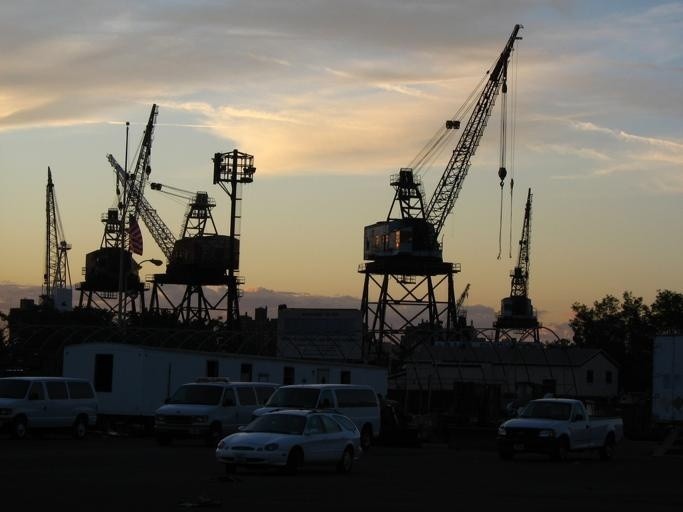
xmin=129 ymin=213 xmax=143 ymax=256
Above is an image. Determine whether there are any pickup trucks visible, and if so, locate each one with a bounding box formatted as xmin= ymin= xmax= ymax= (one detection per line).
xmin=495 ymin=397 xmax=624 ymax=459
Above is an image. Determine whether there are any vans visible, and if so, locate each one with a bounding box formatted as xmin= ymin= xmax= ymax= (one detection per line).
xmin=0 ymin=374 xmax=100 ymax=443
xmin=152 ymin=374 xmax=282 ymax=450
xmin=250 ymin=381 xmax=382 ymax=448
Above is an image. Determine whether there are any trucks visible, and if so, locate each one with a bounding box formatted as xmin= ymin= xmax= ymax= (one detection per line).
xmin=651 ymin=334 xmax=682 ymax=441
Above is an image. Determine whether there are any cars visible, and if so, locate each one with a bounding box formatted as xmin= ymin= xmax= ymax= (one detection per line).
xmin=211 ymin=409 xmax=364 ymax=479
